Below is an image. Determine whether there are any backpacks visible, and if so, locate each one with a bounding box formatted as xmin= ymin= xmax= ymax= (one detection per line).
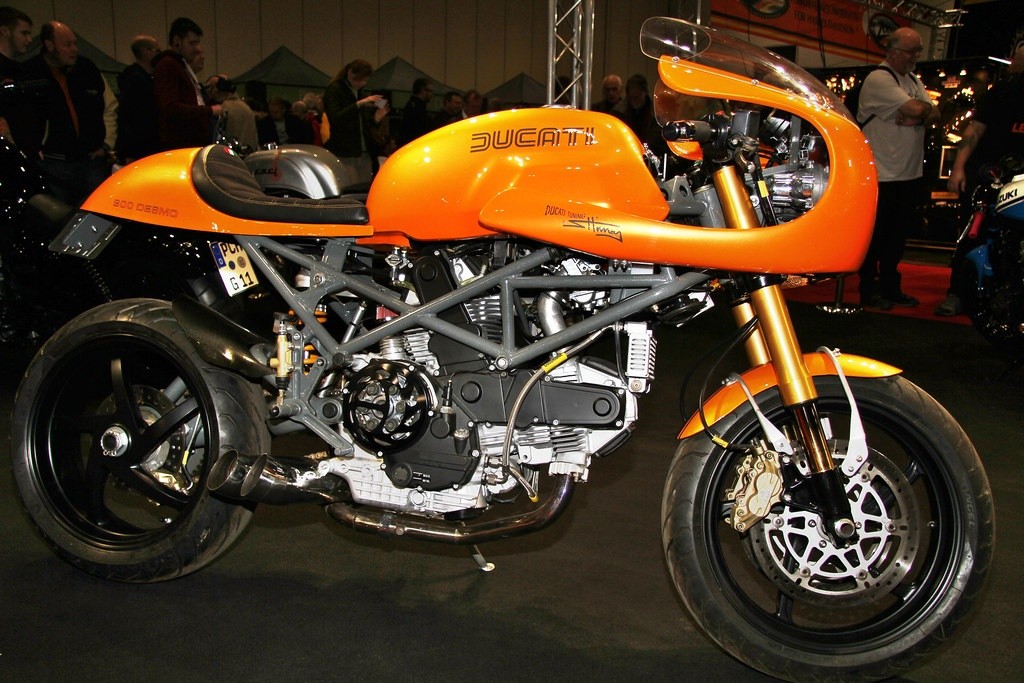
xmin=844 ymin=66 xmax=917 ymax=132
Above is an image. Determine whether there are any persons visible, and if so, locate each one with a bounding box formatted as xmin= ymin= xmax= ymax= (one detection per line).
xmin=150 ymin=16 xmax=225 ymax=150
xmin=553 ymin=73 xmax=671 ymax=156
xmin=114 ymin=34 xmax=160 ymax=166
xmin=857 ymin=27 xmax=941 ymax=311
xmin=204 ymin=59 xmax=525 ymax=183
xmin=1 ymin=3 xmax=112 ymax=207
xmin=933 ymin=32 xmax=1024 ymax=315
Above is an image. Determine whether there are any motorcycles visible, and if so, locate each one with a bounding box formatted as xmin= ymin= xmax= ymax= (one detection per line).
xmin=650 ymin=48 xmax=1024 ymax=359
xmin=8 ymin=14 xmax=999 ymax=683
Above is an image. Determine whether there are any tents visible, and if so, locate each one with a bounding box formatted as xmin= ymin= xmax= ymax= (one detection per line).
xmin=13 ymin=19 xmax=128 ymax=98
xmin=226 ymin=45 xmax=337 ymax=108
xmin=353 ymin=56 xmax=468 ymax=111
xmin=485 ymin=71 xmax=549 ymax=105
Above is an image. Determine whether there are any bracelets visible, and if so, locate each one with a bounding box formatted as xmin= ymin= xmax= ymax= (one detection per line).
xmin=917 ymin=118 xmax=925 ymax=125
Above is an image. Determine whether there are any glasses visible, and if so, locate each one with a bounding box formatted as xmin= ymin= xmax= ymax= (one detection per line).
xmin=891 ymin=46 xmax=924 ymax=55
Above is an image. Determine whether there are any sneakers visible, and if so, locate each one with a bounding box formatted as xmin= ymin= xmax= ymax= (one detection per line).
xmin=886 ymin=292 xmax=919 ymax=307
xmin=934 ymin=292 xmax=963 ymax=316
xmin=860 ymin=294 xmax=893 ymax=310
